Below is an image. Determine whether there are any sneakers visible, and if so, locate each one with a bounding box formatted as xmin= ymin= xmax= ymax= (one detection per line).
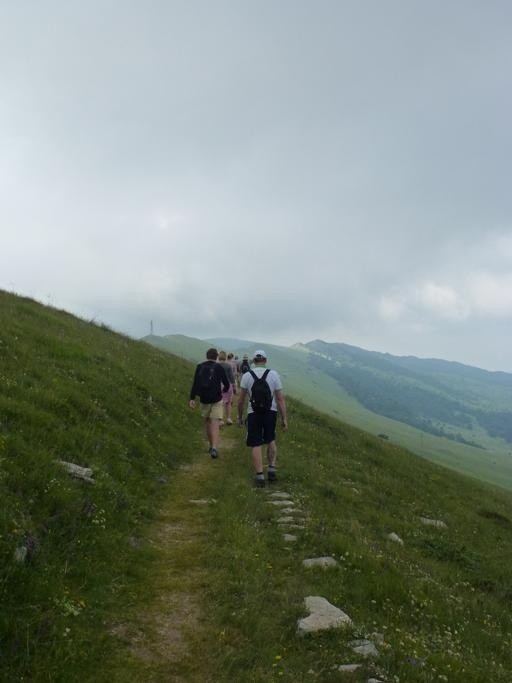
xmin=256 ymin=478 xmax=265 ymax=488
xmin=208 ymin=446 xmax=219 ymax=458
xmin=268 ymin=471 xmax=275 ymax=480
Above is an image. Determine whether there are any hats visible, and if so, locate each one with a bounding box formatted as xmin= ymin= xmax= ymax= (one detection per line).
xmin=255 ymin=350 xmax=266 ymax=359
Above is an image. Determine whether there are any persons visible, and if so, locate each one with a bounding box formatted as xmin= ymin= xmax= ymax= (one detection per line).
xmin=189 ymin=348 xmax=230 ymax=458
xmin=237 ymin=349 xmax=288 ymax=488
xmin=218 ymin=347 xmax=256 ymax=427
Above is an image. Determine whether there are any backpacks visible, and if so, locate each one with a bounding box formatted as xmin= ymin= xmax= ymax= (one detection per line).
xmin=199 ymin=361 xmax=221 ymax=402
xmin=247 ymin=368 xmax=272 ymax=411
